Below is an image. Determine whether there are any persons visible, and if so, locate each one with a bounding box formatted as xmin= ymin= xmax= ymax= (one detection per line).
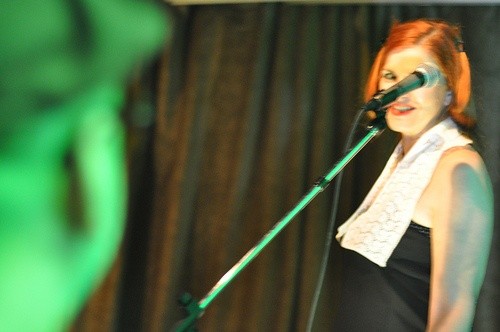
xmin=334 ymin=19 xmax=495 ymax=331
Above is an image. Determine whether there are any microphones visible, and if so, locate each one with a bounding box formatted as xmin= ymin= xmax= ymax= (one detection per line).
xmin=363 ymin=64 xmax=441 ymax=111
